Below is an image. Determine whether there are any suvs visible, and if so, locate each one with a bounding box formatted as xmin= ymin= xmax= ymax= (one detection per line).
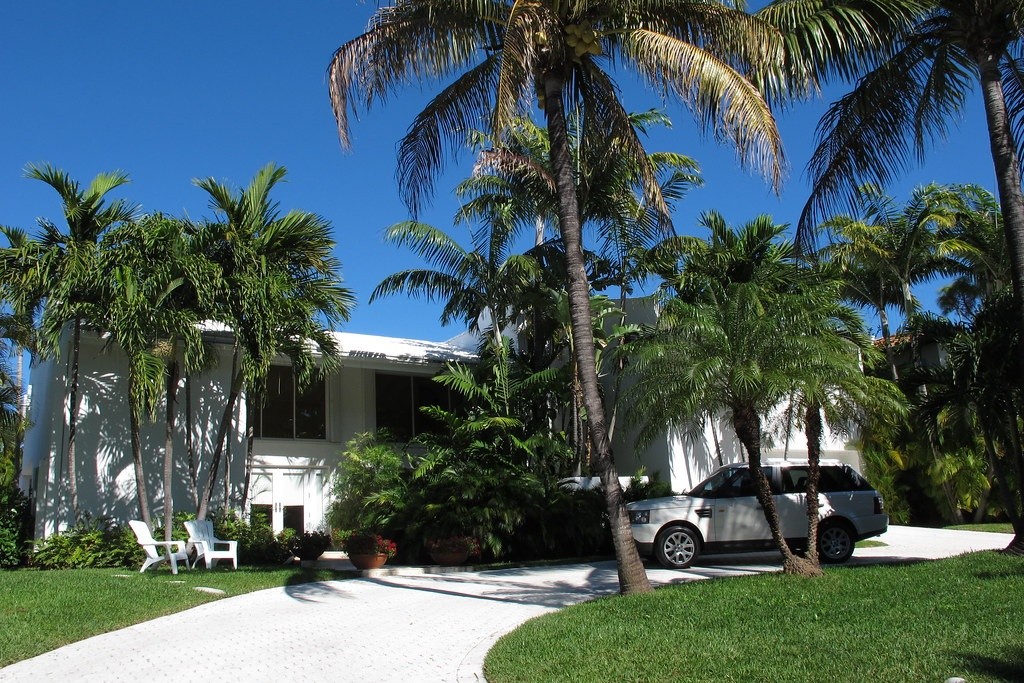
xmin=625 ymin=457 xmax=891 ymax=570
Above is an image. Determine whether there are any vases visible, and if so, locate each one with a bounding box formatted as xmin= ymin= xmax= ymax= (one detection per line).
xmin=351 ymin=553 xmax=388 ymax=569
xmin=294 ymin=549 xmax=325 ymax=560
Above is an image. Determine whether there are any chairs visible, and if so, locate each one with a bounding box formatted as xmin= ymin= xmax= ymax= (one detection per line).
xmin=796 ymin=476 xmax=808 ymax=488
xmin=183 ymin=520 xmax=238 ymax=569
xmin=128 ymin=519 xmax=190 ymax=575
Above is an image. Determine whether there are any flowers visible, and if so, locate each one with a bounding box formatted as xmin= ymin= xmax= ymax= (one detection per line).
xmin=342 ymin=529 xmax=397 ymax=557
xmin=292 ymin=530 xmax=332 ymax=553
